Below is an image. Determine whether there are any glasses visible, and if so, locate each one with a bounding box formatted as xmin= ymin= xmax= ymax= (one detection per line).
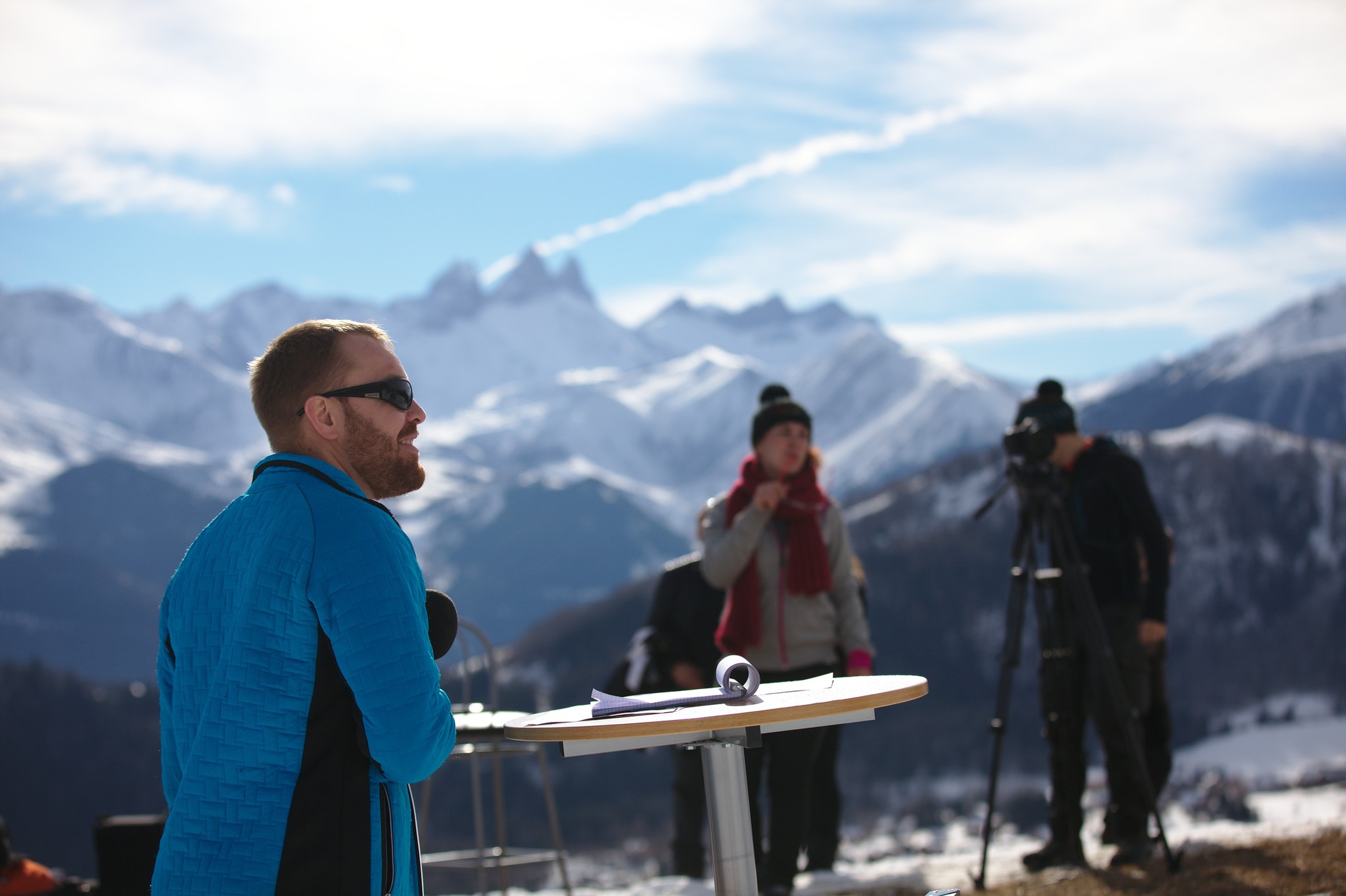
xmin=296 ymin=377 xmax=413 ymax=411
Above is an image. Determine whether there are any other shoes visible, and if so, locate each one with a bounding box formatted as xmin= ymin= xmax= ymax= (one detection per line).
xmin=1024 ymin=836 xmax=1087 ymax=870
xmin=1110 ymin=834 xmax=1152 ymax=867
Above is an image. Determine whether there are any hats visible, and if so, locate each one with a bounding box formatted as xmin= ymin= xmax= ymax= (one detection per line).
xmin=1015 ymin=381 xmax=1075 ymax=436
xmin=750 ymin=385 xmax=812 ymax=447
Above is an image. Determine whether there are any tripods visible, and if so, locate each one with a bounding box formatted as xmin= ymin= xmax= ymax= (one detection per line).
xmin=966 ymin=459 xmax=1183 ymax=886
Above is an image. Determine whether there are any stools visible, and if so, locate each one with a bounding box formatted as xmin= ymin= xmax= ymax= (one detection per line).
xmin=407 ymin=621 xmax=577 ymax=895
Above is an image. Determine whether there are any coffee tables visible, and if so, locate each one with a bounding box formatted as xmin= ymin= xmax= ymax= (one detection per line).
xmin=504 ymin=674 xmax=929 ymax=895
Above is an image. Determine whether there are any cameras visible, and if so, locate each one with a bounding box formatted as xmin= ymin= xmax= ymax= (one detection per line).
xmin=1003 ymin=420 xmax=1056 ymax=457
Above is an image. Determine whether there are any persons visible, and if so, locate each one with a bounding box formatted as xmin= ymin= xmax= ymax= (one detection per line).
xmin=1218 ymin=777 xmax=1251 ymax=823
xmin=1014 ymin=379 xmax=1176 ymax=872
xmin=791 ymin=546 xmax=868 ymax=878
xmin=912 ymin=773 xmax=943 ymax=828
xmin=1177 ymin=767 xmax=1227 ymax=824
xmin=608 ymin=494 xmax=767 ymax=880
xmin=1009 ymin=773 xmax=1047 ymax=836
xmin=1100 ymin=515 xmax=1173 ymax=844
xmin=149 ymin=318 xmax=459 ymax=895
xmin=699 ymin=382 xmax=877 ymax=895
